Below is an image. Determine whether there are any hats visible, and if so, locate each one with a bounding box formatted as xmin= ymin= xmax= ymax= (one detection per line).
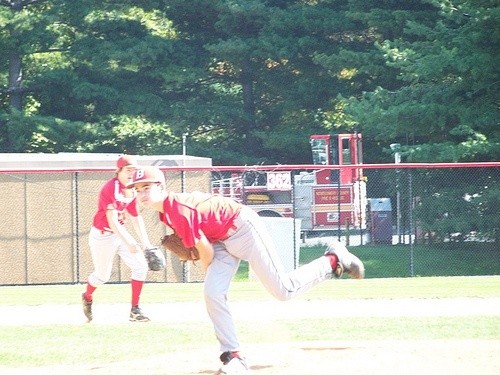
xmin=116 ymin=155 xmax=135 ymax=168
xmin=126 ymin=166 xmax=164 ymax=189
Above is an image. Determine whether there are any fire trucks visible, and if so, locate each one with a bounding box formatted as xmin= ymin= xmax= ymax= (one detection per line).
xmin=208 ymin=131 xmax=366 ymax=230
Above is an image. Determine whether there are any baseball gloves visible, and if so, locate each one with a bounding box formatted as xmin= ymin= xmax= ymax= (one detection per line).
xmin=160 ymin=234 xmax=200 ymax=260
xmin=144 ymin=246 xmax=166 ymax=271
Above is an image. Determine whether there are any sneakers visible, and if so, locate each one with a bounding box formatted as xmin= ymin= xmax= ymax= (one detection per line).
xmin=82 ymin=293 xmax=93 ymax=321
xmin=215 ymin=351 xmax=248 ymax=375
xmin=129 ymin=308 xmax=150 ymax=322
xmin=324 ymin=239 xmax=365 ymax=279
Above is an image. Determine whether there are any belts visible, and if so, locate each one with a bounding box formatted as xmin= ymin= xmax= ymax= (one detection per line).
xmin=219 ymin=206 xmax=249 ymax=241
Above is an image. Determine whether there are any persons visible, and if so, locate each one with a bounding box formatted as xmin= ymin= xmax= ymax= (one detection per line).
xmin=126 ymin=167 xmax=365 ymax=375
xmin=82 ymin=155 xmax=165 ymax=323
